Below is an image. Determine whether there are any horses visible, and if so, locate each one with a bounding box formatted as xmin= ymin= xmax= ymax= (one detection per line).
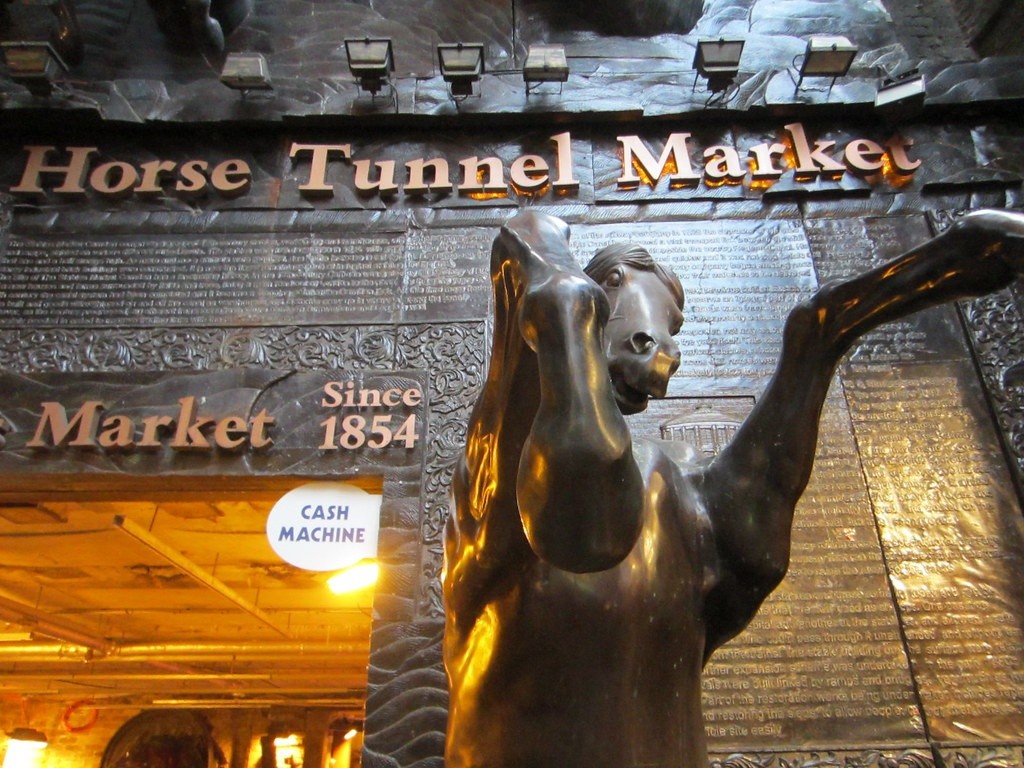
xmin=433 ymin=207 xmax=1024 ymax=767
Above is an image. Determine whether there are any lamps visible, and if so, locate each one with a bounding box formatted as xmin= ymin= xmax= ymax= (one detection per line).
xmin=436 ymin=42 xmax=486 ymax=108
xmin=218 ymin=51 xmax=275 ymax=99
xmin=874 ymin=67 xmax=927 ymax=111
xmin=693 ymin=36 xmax=746 ymax=105
xmin=522 ymin=42 xmax=569 ymax=95
xmin=793 ymin=35 xmax=860 ymax=87
xmin=343 ymin=36 xmax=398 ymax=104
xmin=0 ymin=40 xmax=71 ymax=97
